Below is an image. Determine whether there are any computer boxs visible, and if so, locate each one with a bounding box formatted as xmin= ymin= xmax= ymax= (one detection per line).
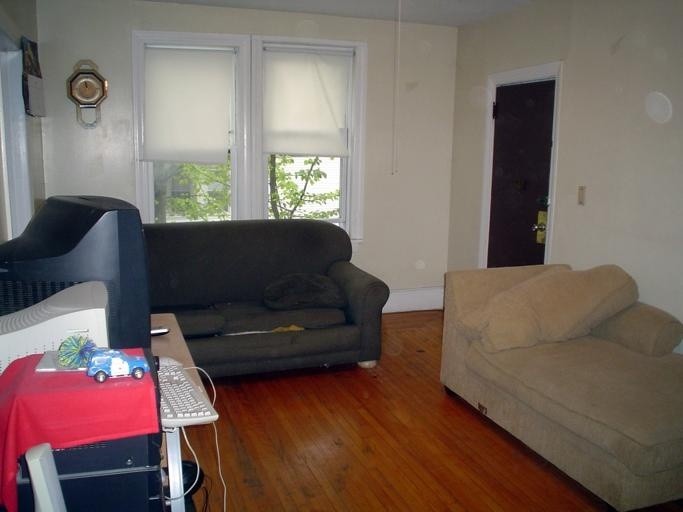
xmin=18 ymin=433 xmax=162 ymax=511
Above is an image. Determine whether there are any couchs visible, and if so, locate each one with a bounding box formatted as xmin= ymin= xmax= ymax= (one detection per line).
xmin=140 ymin=219 xmax=389 ymax=379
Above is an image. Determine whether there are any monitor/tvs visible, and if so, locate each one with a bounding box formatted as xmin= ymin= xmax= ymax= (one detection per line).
xmin=0 ymin=195 xmax=151 ymax=349
xmin=0 ymin=279 xmax=113 ymax=375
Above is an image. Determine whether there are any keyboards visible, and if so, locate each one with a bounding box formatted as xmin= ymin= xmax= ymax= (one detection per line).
xmin=157 ymin=355 xmax=219 ymax=427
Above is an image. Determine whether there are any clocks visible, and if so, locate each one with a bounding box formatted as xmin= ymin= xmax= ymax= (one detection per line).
xmin=66 ymin=59 xmax=108 ymax=129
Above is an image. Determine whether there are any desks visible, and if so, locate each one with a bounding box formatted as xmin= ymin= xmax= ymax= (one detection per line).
xmin=150 ymin=313 xmax=214 ymax=512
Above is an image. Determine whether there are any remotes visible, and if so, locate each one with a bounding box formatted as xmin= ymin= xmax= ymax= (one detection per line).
xmin=152 ymin=326 xmax=170 ymax=338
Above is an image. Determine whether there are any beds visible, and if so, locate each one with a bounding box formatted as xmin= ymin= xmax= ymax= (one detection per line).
xmin=440 ymin=264 xmax=683 ymax=512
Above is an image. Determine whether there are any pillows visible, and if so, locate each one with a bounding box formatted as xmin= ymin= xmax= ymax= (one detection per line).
xmin=481 ymin=264 xmax=638 ymax=352
xmin=264 ymin=272 xmax=347 ymax=311
